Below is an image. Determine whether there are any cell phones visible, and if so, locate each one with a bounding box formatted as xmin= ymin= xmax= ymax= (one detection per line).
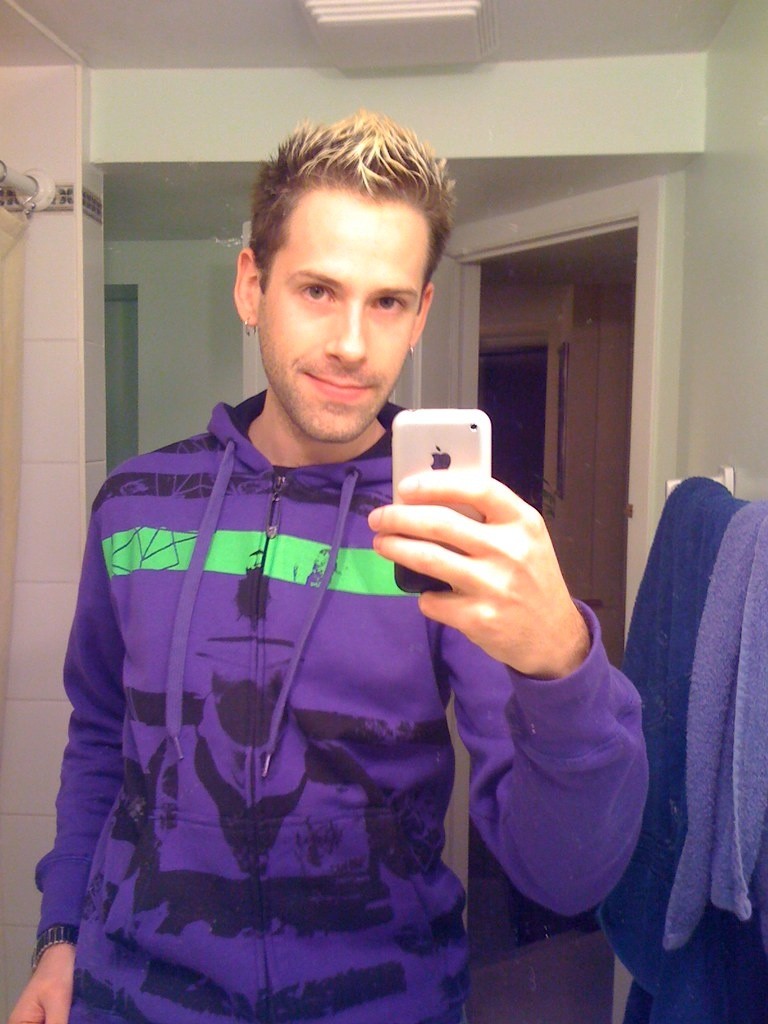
xmin=392 ymin=409 xmax=492 ymax=593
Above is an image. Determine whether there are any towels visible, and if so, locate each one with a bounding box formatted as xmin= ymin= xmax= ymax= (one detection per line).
xmin=593 ymin=475 xmax=767 ymax=1023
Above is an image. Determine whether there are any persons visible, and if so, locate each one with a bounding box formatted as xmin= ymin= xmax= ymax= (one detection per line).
xmin=7 ymin=111 xmax=649 ymax=1024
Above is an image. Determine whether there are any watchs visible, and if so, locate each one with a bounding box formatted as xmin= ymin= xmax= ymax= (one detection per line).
xmin=29 ymin=926 xmax=80 ymax=972
